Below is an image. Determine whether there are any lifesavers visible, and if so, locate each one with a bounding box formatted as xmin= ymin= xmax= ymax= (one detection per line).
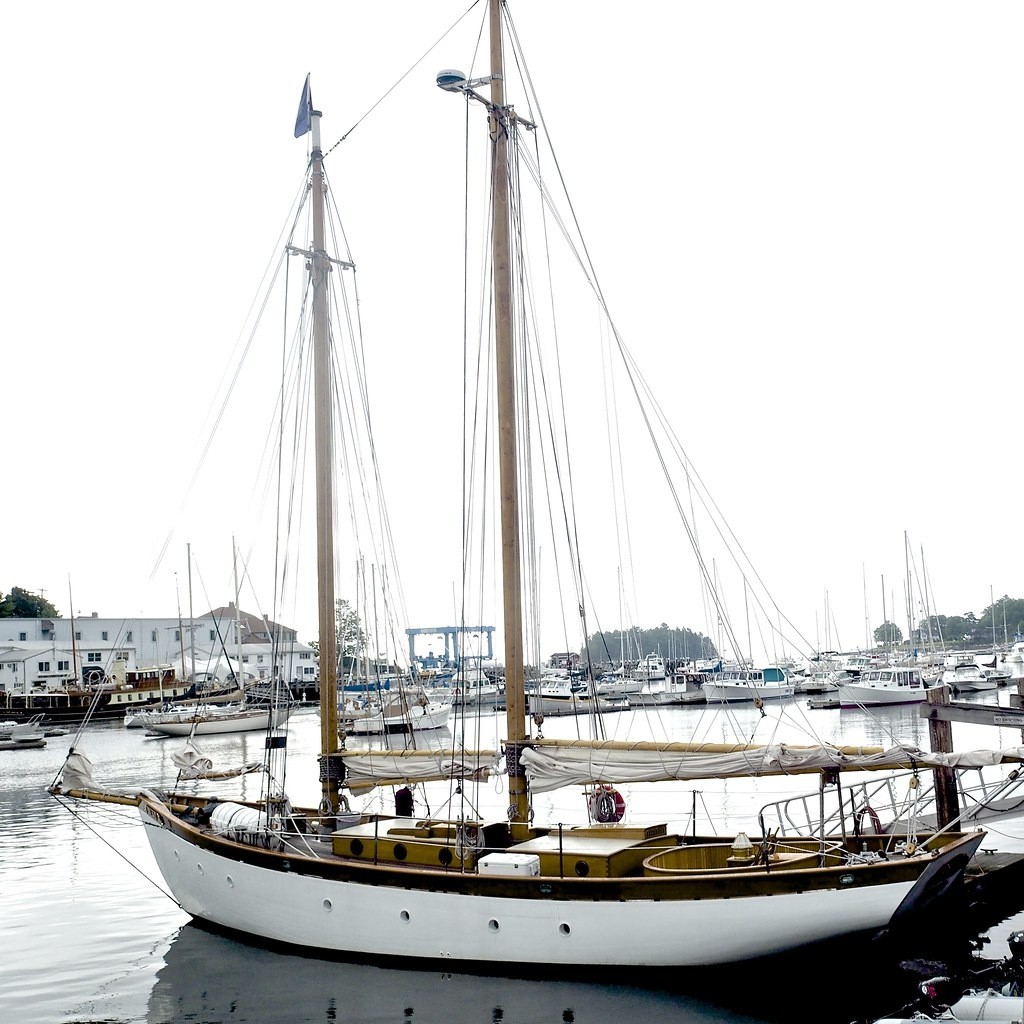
xmin=590 ymin=786 xmax=626 ymax=821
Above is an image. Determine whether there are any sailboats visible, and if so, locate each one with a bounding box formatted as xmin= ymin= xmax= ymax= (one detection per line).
xmin=0 ymin=0 xmax=1024 ymax=973
